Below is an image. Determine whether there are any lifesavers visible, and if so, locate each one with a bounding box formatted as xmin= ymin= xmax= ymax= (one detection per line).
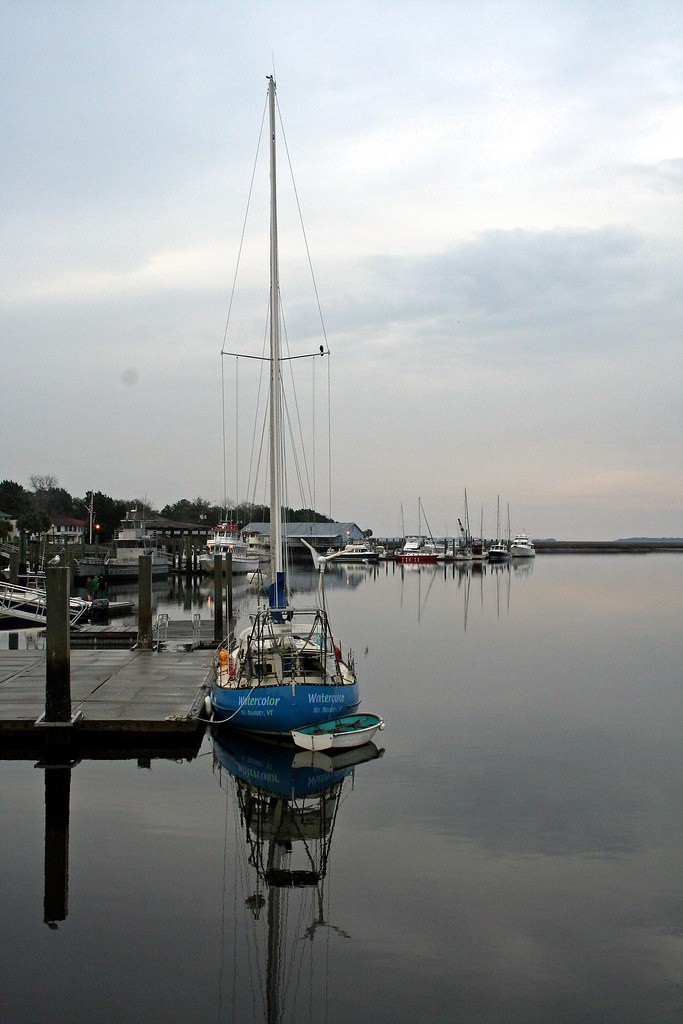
xmin=334 ymin=647 xmax=345 ymax=663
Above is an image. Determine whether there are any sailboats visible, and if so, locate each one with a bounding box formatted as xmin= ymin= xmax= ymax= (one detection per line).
xmin=209 ymin=74 xmax=362 ymax=731
xmin=212 ymin=737 xmax=351 ymax=1024
xmin=378 ymin=488 xmax=510 ymax=562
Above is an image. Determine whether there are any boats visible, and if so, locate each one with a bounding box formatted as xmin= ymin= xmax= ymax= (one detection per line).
xmin=74 ymin=505 xmax=173 ymax=577
xmin=199 ymin=523 xmax=260 ymax=572
xmin=291 ymin=741 xmax=379 ymax=772
xmin=510 ymin=529 xmax=536 ymax=557
xmin=182 ymin=548 xmax=207 ymax=564
xmin=395 ymin=554 xmax=440 ymax=564
xmin=291 ymin=713 xmax=386 ymax=751
xmin=325 ymin=544 xmax=379 ymax=562
xmin=243 ymin=529 xmax=272 ymax=562
